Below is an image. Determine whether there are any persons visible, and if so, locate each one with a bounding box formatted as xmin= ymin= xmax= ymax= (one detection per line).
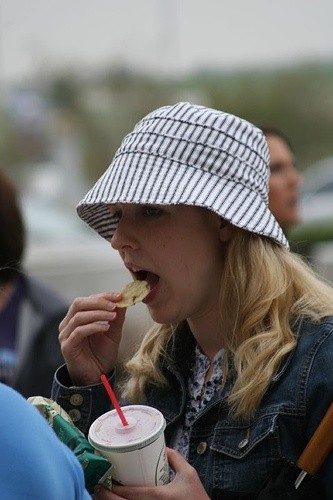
xmin=258 ymin=126 xmax=301 ymax=240
xmin=45 ymin=101 xmax=333 ymax=500
xmin=0 ymin=170 xmax=74 ymax=400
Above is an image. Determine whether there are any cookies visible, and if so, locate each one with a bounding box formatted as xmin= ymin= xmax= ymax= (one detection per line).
xmin=114 ymin=279 xmax=150 ymax=309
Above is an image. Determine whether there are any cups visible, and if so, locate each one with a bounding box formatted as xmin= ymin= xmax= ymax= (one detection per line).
xmin=88 ymin=405 xmax=171 ymax=488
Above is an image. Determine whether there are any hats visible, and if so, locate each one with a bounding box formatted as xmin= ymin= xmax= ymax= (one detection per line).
xmin=77 ymin=101 xmax=291 ymax=247
